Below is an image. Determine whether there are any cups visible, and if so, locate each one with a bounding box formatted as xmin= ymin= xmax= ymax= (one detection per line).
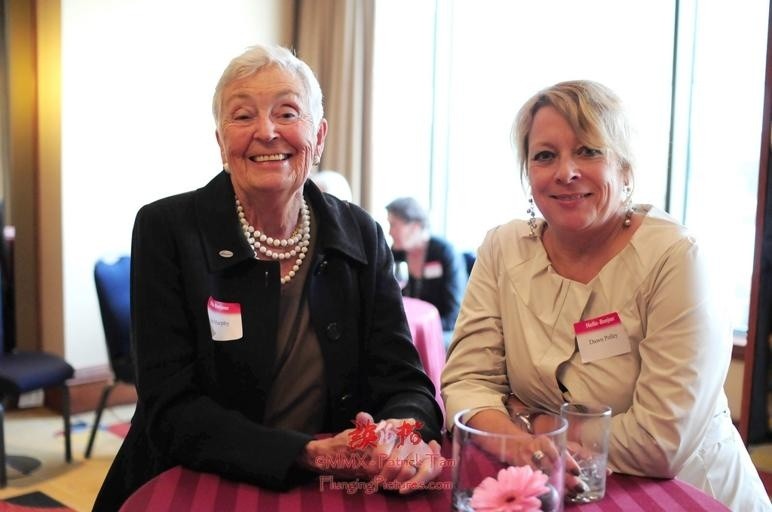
xmin=392 ymin=261 xmax=409 ymax=290
xmin=450 ymin=404 xmax=569 ymax=512
xmin=561 ymin=402 xmax=612 ymax=508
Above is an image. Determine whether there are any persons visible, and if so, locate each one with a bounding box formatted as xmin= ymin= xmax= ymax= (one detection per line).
xmin=310 ymin=170 xmax=352 ymax=204
xmin=440 ymin=80 xmax=772 ymax=512
xmin=385 ymin=197 xmax=469 ymax=331
xmin=93 ymin=44 xmax=444 ymax=512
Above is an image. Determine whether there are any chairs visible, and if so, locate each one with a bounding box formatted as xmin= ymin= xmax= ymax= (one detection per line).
xmin=0 ymin=198 xmax=76 ymax=488
xmin=83 ymin=256 xmax=136 ymax=457
xmin=462 ymin=252 xmax=476 ymax=276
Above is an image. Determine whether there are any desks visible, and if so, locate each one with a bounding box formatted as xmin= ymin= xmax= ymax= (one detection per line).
xmin=115 ymin=434 xmax=730 ymax=512
xmin=401 ymin=295 xmax=447 ymax=434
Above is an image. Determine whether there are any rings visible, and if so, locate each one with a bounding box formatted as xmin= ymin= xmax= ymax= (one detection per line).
xmin=532 ymin=449 xmax=545 ymax=463
xmin=409 ymin=461 xmax=418 ymax=469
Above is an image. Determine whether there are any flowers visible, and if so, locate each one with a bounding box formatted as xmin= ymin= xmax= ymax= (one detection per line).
xmin=470 ymin=463 xmax=551 ymax=512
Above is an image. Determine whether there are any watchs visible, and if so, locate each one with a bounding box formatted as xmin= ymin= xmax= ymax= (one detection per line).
xmin=513 ymin=411 xmax=549 ymax=434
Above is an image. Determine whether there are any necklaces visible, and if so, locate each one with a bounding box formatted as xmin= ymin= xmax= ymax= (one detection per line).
xmin=234 ymin=192 xmax=311 ymax=286
